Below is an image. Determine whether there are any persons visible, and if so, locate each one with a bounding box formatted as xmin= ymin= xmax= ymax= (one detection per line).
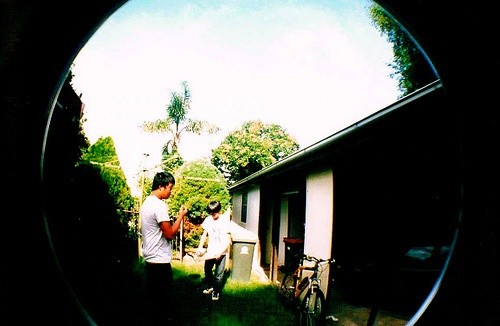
xmin=196 ymin=201 xmax=232 ymax=300
xmin=140 ymin=172 xmax=187 ymax=280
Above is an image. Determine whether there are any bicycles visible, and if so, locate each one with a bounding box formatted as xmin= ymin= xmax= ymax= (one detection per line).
xmin=278 ymin=253 xmax=336 ymax=326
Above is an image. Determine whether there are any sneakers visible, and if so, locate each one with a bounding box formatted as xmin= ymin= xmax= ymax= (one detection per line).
xmin=202 ymin=287 xmax=214 ymax=295
xmin=212 ymin=291 xmax=219 ymax=301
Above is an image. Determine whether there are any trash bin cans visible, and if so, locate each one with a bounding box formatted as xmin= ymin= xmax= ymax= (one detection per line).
xmin=231 ymin=240 xmax=256 ymax=281
